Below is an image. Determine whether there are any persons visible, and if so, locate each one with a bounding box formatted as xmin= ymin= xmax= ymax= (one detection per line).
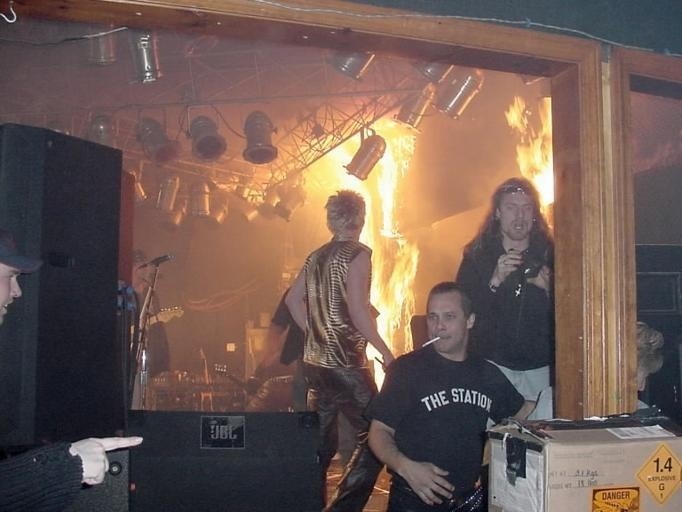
xmin=284 ymin=186 xmax=394 ymax=466
xmin=246 ymin=324 xmax=291 ymax=395
xmin=362 ymin=280 xmax=535 ymax=512
xmin=527 ymin=322 xmax=662 ymax=420
xmin=0 ymin=234 xmax=144 ymax=512
xmin=133 ymin=251 xmax=170 ymax=410
xmin=457 ymin=174 xmax=555 ymax=420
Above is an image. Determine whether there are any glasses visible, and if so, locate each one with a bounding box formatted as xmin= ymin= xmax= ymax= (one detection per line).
xmin=499 ymin=184 xmax=532 ymax=196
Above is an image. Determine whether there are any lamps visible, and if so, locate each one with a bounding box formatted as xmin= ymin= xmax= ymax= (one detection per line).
xmin=126 ymin=29 xmax=162 ymax=86
xmin=126 ymin=167 xmax=263 ymax=222
xmin=256 ymin=181 xmax=310 ymax=222
xmin=390 ymin=88 xmax=435 ymax=137
xmin=80 ymin=107 xmax=116 ymax=146
xmin=184 ymin=109 xmax=231 ymax=162
xmin=239 ymin=108 xmax=280 ymax=165
xmin=435 ymin=64 xmax=484 ymax=125
xmin=337 ymin=132 xmax=390 ymax=183
xmin=334 ymin=48 xmax=378 ymax=83
xmin=49 ymin=114 xmax=70 ymax=138
xmin=129 ymin=108 xmax=184 ymax=171
xmin=64 ymin=25 xmax=117 ymax=79
xmin=419 ymin=47 xmax=457 ymax=79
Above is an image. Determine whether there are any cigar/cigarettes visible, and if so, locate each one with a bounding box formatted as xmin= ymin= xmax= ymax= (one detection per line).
xmin=422 ymin=337 xmax=440 ymax=348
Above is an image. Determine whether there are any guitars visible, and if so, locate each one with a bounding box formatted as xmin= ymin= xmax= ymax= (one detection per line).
xmin=200 ymin=349 xmax=214 ymax=412
xmin=130 ymin=306 xmax=184 ymax=337
xmin=212 ymin=363 xmax=263 ymax=396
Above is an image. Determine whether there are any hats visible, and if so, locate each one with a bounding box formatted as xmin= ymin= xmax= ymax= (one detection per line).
xmin=1 ymin=232 xmax=43 ymax=275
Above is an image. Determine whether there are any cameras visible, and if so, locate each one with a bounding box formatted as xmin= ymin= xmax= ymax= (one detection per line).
xmin=512 ymin=246 xmax=545 ymax=279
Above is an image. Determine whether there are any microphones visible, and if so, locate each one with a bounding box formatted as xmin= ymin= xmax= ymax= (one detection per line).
xmin=138 ymin=251 xmax=177 ymax=269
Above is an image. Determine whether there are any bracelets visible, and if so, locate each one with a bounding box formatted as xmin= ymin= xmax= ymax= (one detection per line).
xmin=488 ymin=282 xmax=498 ymax=290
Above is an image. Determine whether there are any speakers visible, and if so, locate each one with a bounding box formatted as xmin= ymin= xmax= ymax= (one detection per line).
xmin=65 ymin=448 xmax=129 ymax=512
xmin=127 ymin=410 xmax=324 ymax=512
xmin=0 ymin=121 xmax=123 ymax=448
